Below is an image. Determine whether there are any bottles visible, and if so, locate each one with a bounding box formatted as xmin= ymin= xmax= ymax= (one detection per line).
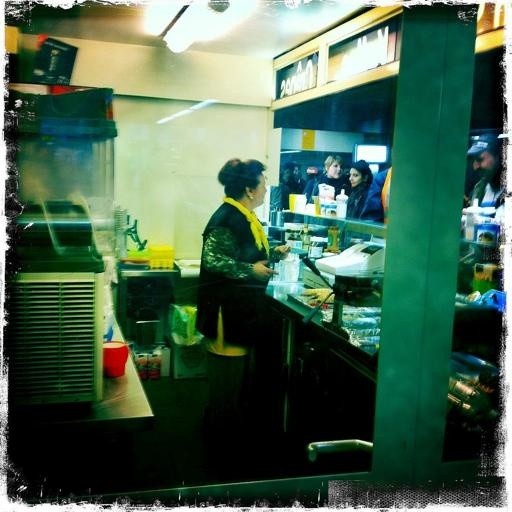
xmin=303 ymin=224 xmax=309 ymax=245
xmin=336 ymin=189 xmax=348 ymax=219
xmin=295 ymin=230 xmax=302 ymax=249
xmin=287 ymin=230 xmax=295 ymax=249
xmin=294 ymin=194 xmax=306 ymax=211
xmin=327 ymin=226 xmax=340 ymax=251
xmin=314 ymin=197 xmax=320 ymax=215
xmin=289 ymin=194 xmax=295 ymax=211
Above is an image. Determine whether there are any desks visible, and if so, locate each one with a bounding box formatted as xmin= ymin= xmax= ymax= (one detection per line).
xmin=117 ymin=264 xmax=180 ymax=338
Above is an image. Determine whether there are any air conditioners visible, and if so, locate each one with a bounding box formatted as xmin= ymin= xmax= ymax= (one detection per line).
xmin=7 ymin=271 xmax=104 ymax=405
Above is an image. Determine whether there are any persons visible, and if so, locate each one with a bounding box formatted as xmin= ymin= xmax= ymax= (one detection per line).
xmin=270 ymin=162 xmax=306 ymax=209
xmin=302 ymin=155 xmax=351 ymax=205
xmin=358 ymin=166 xmax=392 ymax=226
xmin=464 ymin=133 xmax=506 ymax=217
xmin=188 ymin=160 xmax=291 ymax=441
xmin=344 ymin=160 xmax=373 ymax=220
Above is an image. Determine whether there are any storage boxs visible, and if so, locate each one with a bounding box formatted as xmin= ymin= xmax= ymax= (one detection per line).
xmin=171 ymin=340 xmax=209 ymax=380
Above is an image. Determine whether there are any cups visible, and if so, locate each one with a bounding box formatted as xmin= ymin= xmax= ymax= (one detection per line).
xmin=103 ymin=340 xmax=129 ymax=379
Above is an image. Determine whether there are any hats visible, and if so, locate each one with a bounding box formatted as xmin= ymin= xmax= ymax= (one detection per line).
xmin=467 ymin=134 xmax=498 ymax=155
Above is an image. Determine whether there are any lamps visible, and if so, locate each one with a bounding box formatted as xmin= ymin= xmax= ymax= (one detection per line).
xmin=161 ymin=1 xmax=232 ymax=54
